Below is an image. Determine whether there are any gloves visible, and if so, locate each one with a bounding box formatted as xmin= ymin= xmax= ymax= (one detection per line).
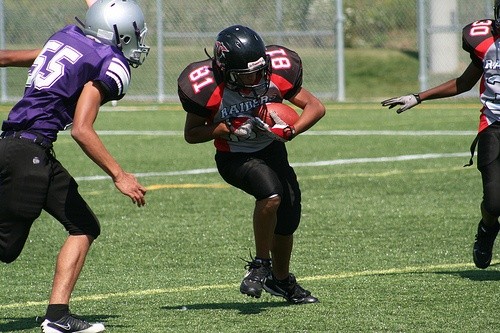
xmin=228 ymin=114 xmax=259 ymax=141
xmin=251 ymin=111 xmax=295 ymax=142
xmin=381 ymin=94 xmax=421 ymax=114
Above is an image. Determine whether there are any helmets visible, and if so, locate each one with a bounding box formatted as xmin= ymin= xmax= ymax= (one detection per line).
xmin=214 ymin=25 xmax=267 ymax=82
xmin=83 ymin=0 xmax=145 ymax=61
xmin=494 ymin=0 xmax=500 ymax=19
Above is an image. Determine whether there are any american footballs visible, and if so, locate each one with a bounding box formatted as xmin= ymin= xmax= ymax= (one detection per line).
xmin=253 ymin=101 xmax=300 ymax=128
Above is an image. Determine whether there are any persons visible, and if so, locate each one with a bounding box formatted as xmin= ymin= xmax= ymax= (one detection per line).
xmin=381 ymin=0 xmax=500 ymax=269
xmin=179 ymin=25 xmax=326 ymax=302
xmin=0 ymin=0 xmax=150 ymax=333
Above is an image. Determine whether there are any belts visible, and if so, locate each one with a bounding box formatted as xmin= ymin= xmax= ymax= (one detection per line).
xmin=4 ymin=131 xmax=37 ymax=141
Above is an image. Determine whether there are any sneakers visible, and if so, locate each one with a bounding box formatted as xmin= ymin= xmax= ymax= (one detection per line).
xmin=41 ymin=311 xmax=106 ymax=333
xmin=262 ymin=272 xmax=320 ymax=303
xmin=472 ymin=219 xmax=500 ymax=269
xmin=240 ymin=248 xmax=271 ymax=298
